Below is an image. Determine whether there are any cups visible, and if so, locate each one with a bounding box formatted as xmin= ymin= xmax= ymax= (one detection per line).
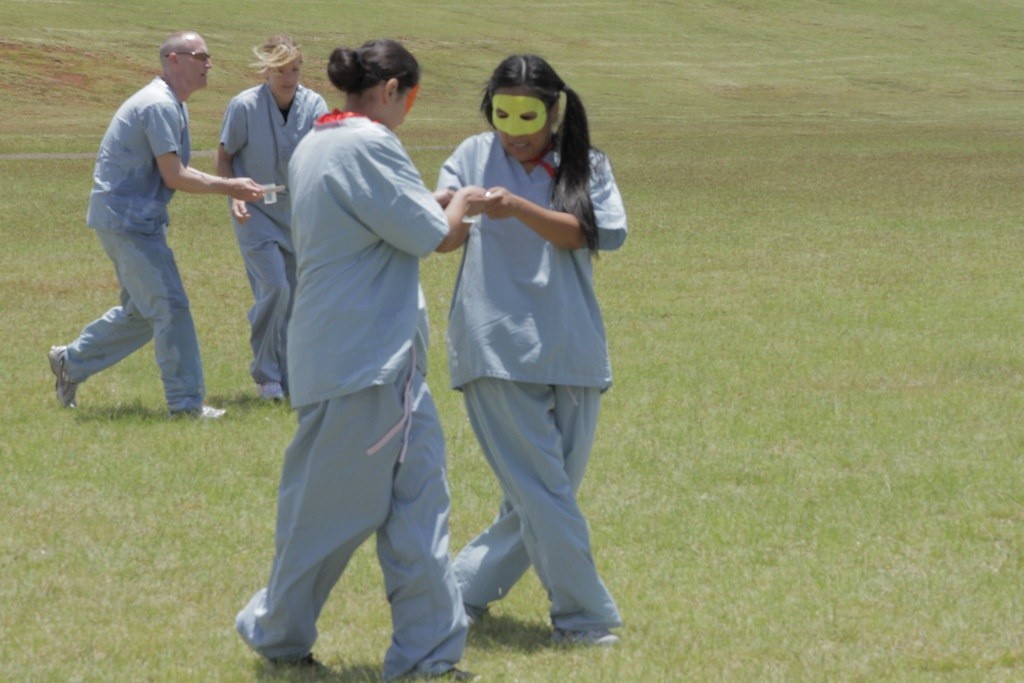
xmin=462 ymin=213 xmax=481 ymax=223
xmin=261 ymin=183 xmax=276 ymax=204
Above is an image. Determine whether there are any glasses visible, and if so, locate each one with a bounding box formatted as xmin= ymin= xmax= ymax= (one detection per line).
xmin=166 ymin=50 xmax=211 ymax=63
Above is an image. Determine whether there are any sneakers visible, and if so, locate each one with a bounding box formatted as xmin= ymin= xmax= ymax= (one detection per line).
xmin=49 ymin=346 xmax=78 ymax=411
xmin=254 ymin=381 xmax=285 ymax=405
xmin=165 ymin=404 xmax=227 ymax=422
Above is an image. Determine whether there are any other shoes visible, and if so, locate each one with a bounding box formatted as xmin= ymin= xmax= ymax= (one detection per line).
xmin=236 ymin=612 xmax=323 ymax=673
xmin=385 ymin=663 xmax=479 ymax=683
xmin=463 ymin=600 xmax=490 ymax=631
xmin=549 ymin=627 xmax=619 ymax=644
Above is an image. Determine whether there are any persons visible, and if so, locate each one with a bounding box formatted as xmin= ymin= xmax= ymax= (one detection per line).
xmin=233 ymin=38 xmax=491 ymax=683
xmin=214 ymin=39 xmax=329 ymax=404
xmin=438 ymin=54 xmax=628 ymax=645
xmin=47 ymin=32 xmax=283 ymax=421
